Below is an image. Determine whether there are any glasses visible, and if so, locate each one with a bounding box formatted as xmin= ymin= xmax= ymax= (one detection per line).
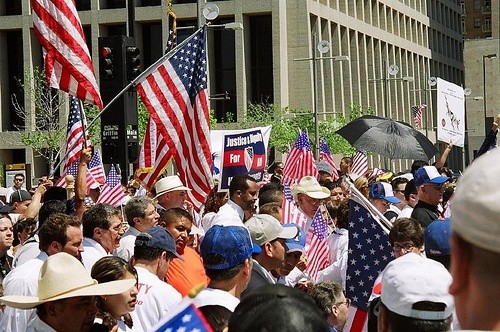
xmin=393 ymin=189 xmax=405 ymax=195
xmin=334 ymin=298 xmax=352 ymax=308
xmin=393 ymin=243 xmax=415 ymax=252
xmin=381 ymin=202 xmax=391 ymax=207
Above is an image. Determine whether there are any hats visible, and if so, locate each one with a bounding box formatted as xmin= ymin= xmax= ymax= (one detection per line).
xmin=0 ymin=199 xmax=14 ymax=213
xmin=0 ymin=252 xmax=136 ymax=310
xmin=200 ymin=225 xmax=262 ymax=270
xmin=282 ymin=223 xmax=306 ymax=253
xmin=414 ymin=166 xmax=450 ymax=188
xmin=381 ymin=253 xmax=454 ymax=319
xmin=288 ymin=176 xmax=331 ymax=199
xmin=314 ymin=160 xmax=333 ymax=175
xmin=135 ymin=226 xmax=185 ymax=262
xmin=450 ymin=147 xmax=500 ymax=254
xmin=368 ymin=182 xmax=401 ymax=204
xmin=424 ymin=218 xmax=451 ymax=256
xmin=244 ymin=213 xmax=298 ymax=246
xmin=151 ymin=176 xmax=192 ymax=200
xmin=229 ymin=284 xmax=331 ymax=332
xmin=8 ymin=190 xmax=33 ymax=204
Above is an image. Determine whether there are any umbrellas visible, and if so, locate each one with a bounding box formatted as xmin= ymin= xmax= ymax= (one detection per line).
xmin=334 ymin=115 xmax=440 ymax=163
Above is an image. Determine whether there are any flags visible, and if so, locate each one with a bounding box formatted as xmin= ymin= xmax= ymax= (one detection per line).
xmin=131 ymin=23 xmax=219 ymax=213
xmin=281 ymin=180 xmax=308 ymax=231
xmin=30 ymin=0 xmax=104 ymax=111
xmin=95 ymin=163 xmax=127 ymax=208
xmin=303 ymin=203 xmax=335 ymax=284
xmin=280 ymin=127 xmax=321 ymax=187
xmin=412 ymin=105 xmax=425 ymax=130
xmin=319 ymin=137 xmax=339 ymax=181
xmin=137 ymin=22 xmax=178 ymax=191
xmin=342 ymin=192 xmax=396 ymax=332
xmin=54 ymin=95 xmax=106 ymax=190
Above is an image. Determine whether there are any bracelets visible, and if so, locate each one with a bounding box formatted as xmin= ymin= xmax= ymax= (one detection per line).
xmin=446 ymin=145 xmax=452 ymax=149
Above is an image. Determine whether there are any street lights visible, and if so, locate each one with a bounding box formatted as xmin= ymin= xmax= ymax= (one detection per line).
xmin=368 ymin=77 xmax=414 ymax=119
xmin=284 ymin=55 xmax=349 ymax=159
xmin=175 ymin=23 xmax=244 ymax=114
xmin=462 ymin=97 xmax=484 ymax=171
xmin=483 ymin=54 xmax=497 ymax=117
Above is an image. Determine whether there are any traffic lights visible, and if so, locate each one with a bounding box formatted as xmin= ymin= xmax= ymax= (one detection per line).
xmin=126 ymin=46 xmax=141 ymax=92
xmin=97 ymin=46 xmax=116 ymax=82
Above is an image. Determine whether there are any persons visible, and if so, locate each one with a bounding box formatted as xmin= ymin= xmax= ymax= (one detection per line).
xmin=447 ymin=146 xmax=500 ymax=332
xmin=0 ymin=156 xmax=500 ymax=332
